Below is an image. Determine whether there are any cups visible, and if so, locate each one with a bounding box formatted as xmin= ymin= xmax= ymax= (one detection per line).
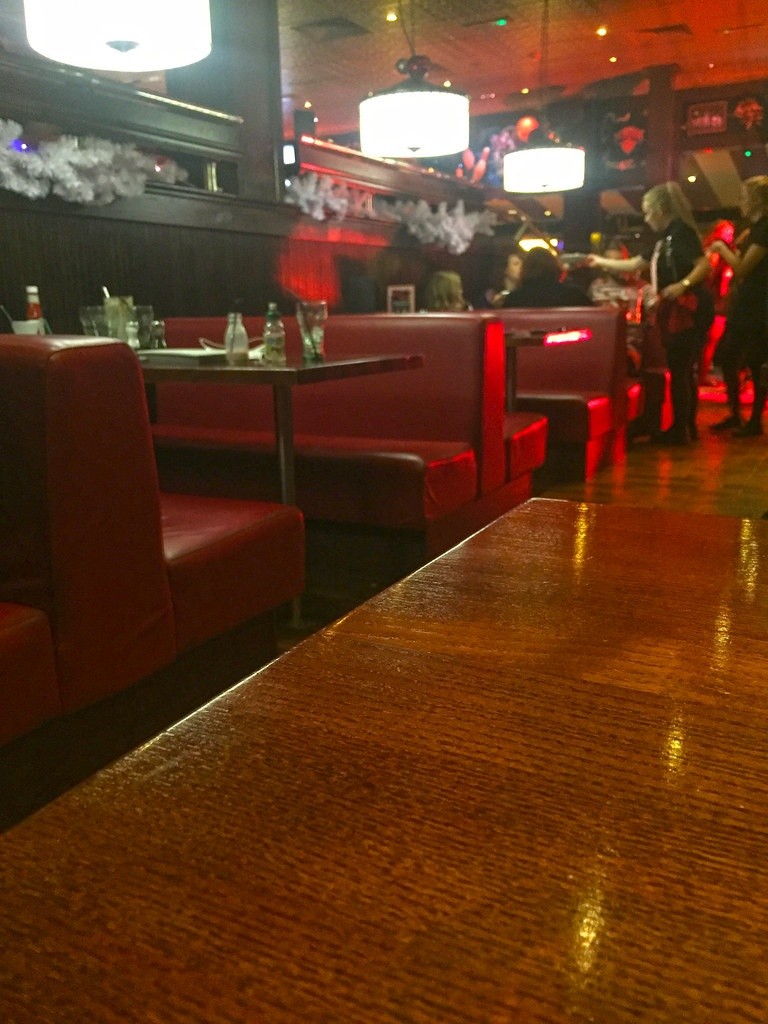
xmin=296 ymin=301 xmax=328 ymax=358
xmin=11 ymin=320 xmax=46 ymax=334
xmin=79 ymin=295 xmax=154 ymax=351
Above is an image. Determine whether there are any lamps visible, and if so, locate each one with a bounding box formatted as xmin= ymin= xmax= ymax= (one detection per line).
xmin=503 ymin=0 xmax=585 ymax=194
xmin=359 ymin=0 xmax=470 ymax=158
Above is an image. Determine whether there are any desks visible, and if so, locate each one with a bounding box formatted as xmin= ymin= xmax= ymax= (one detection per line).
xmin=0 ymin=494 xmax=768 ymax=1024
xmin=139 ymin=355 xmax=424 ymax=638
xmin=507 ymin=326 xmax=593 ymax=412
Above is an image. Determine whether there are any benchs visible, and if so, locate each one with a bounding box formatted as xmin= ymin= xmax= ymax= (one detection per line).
xmin=0 ymin=307 xmax=674 ymax=752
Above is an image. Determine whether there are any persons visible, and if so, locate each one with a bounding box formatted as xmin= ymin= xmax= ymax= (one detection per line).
xmin=422 ymin=175 xmax=768 ymax=446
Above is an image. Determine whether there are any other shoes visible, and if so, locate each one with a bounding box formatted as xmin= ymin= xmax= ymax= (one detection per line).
xmin=712 ymin=412 xmax=743 ymax=432
xmin=732 ymin=415 xmax=765 ymax=437
xmin=652 ymin=422 xmax=698 ymax=446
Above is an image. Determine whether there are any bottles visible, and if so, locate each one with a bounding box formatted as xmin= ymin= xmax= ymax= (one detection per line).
xmin=152 ymin=320 xmax=167 ymax=349
xmin=125 ymin=321 xmax=141 ymax=350
xmin=264 ymin=303 xmax=286 ymax=362
xmin=224 ymin=312 xmax=248 ymax=364
xmin=25 ymin=285 xmax=42 ymax=320
